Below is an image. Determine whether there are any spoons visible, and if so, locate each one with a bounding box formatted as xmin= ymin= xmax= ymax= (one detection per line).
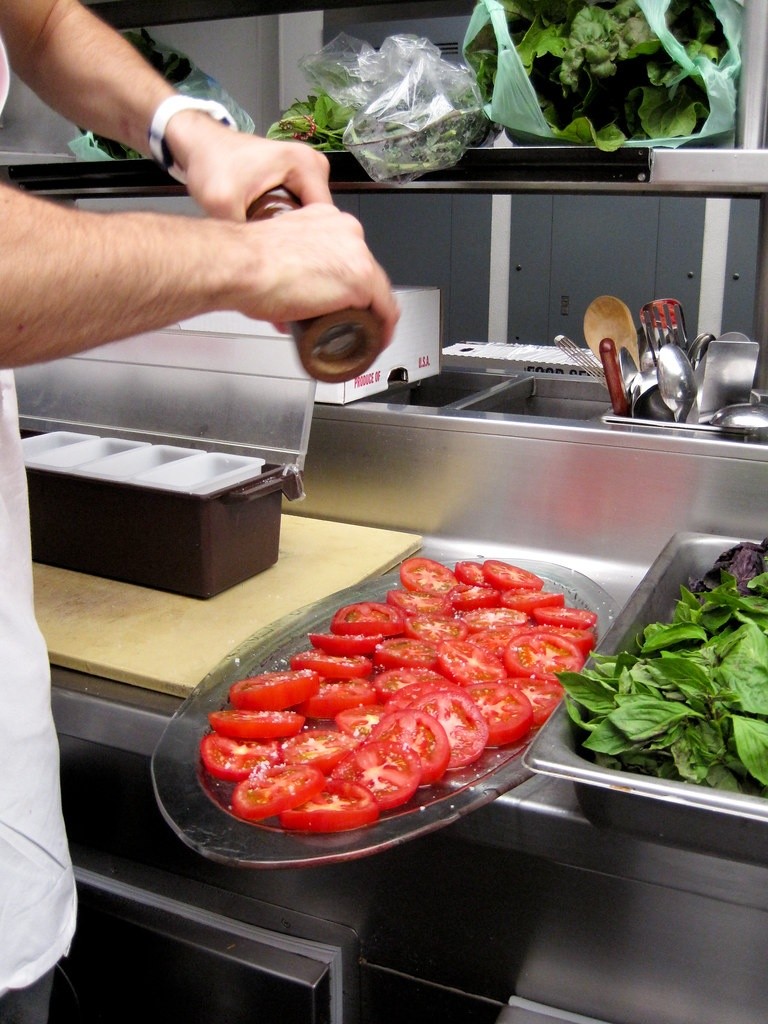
xmin=685 ymin=329 xmax=715 ymax=372
xmin=657 ymin=342 xmax=699 ymax=427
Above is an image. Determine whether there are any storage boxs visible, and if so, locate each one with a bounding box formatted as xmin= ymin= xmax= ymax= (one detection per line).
xmin=179 ymin=285 xmax=446 ymax=405
xmin=13 ymin=326 xmax=319 ymax=601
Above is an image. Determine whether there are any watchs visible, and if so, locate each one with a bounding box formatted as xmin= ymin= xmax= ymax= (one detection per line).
xmin=145 ymin=96 xmax=239 ymax=187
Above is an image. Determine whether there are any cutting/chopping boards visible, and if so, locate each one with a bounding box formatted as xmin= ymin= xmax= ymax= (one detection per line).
xmin=27 ymin=511 xmax=428 ymax=706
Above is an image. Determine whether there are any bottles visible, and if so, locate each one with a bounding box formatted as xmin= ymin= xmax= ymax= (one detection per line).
xmin=247 ymin=188 xmax=387 ymax=384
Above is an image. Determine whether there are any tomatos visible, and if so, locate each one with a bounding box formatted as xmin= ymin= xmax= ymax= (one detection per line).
xmin=199 ymin=557 xmax=597 ymax=834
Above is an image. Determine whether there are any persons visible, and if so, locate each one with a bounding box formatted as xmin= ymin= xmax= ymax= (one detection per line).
xmin=0 ymin=1 xmax=402 ymax=1024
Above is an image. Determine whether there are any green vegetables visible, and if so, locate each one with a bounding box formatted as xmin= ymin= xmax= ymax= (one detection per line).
xmin=553 ymin=532 xmax=767 ymax=798
xmin=77 ymin=0 xmax=730 ymax=180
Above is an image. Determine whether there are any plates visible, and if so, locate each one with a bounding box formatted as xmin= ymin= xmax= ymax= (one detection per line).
xmin=149 ymin=557 xmax=623 ymax=867
